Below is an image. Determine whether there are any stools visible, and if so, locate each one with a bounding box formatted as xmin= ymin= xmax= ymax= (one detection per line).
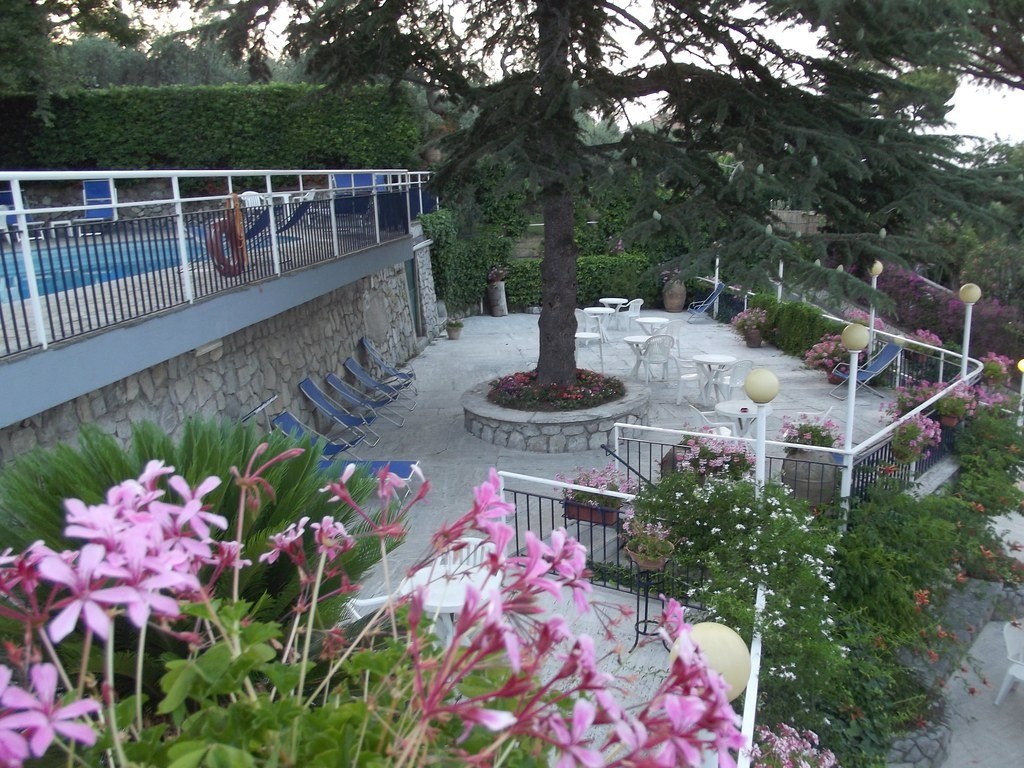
xmin=49 ymin=219 xmax=74 ymax=239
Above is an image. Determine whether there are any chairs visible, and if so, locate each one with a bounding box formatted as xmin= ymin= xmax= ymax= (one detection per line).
xmin=669 ymin=349 xmax=710 ymax=407
xmin=318 ymin=455 xmax=420 ymax=510
xmin=292 ymin=188 xmax=316 ymax=226
xmin=324 ymin=372 xmax=406 ymax=427
xmin=615 ymin=298 xmax=644 ymax=332
xmin=0 ymin=190 xmax=46 ymax=243
xmin=994 ymin=619 xmax=1024 ymax=706
xmin=639 ymin=334 xmax=675 ymax=386
xmin=178 ymin=205 xmax=285 ymax=275
xmin=345 ymin=593 xmax=398 ymax=621
xmin=241 ymin=190 xmax=267 ymax=212
xmin=272 ymin=408 xmax=367 ymax=461
xmin=298 ymin=376 xmax=381 ymax=448
xmin=362 ymin=336 xmax=419 ymax=396
xmin=436 ymin=536 xmax=506 ymax=587
xmin=652 ymin=319 xmax=684 ymax=359
xmin=73 ymin=178 xmax=119 ymax=237
xmin=321 ymin=173 xmax=387 ymax=232
xmin=344 ymin=357 xmax=417 ymax=410
xmin=574 ymin=308 xmax=610 ymax=343
xmin=707 ymin=359 xmax=754 ymax=401
xmin=829 ymin=342 xmax=902 ymax=401
xmin=687 ymin=404 xmax=738 ymax=438
xmin=687 ymin=282 xmax=725 ymax=325
xmin=225 ymin=201 xmax=310 ymax=265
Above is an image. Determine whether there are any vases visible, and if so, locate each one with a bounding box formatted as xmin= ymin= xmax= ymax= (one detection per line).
xmin=525 ymin=306 xmax=543 ymax=314
xmin=425 ymin=148 xmax=442 ymax=162
xmin=623 ymin=535 xmax=674 ymax=571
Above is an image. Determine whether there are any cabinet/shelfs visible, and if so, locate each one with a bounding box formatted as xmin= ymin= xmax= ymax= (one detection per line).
xmin=627 ymin=570 xmax=672 ymax=654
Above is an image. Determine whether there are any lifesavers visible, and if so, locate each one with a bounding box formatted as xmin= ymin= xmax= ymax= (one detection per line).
xmin=208 ymin=217 xmax=242 ymax=278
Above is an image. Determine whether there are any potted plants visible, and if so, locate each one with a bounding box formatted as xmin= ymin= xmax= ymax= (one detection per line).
xmin=445 ymin=317 xmax=464 ymax=340
xmin=728 ymin=306 xmax=778 ymax=347
xmin=801 ymin=333 xmax=870 ymax=384
xmin=486 ymin=264 xmax=508 ymax=316
xmin=932 ymin=383 xmax=978 ymax=427
xmin=660 ymin=267 xmax=688 ymax=313
xmin=777 ymin=412 xmax=845 ymax=504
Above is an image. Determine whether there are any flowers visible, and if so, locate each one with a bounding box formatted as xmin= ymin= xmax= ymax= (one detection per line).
xmin=617 ymin=507 xmax=673 ymax=561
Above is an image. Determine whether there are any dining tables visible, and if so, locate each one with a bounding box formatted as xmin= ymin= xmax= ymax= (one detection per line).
xmin=574 ymin=332 xmax=604 ymax=374
xmin=400 ymin=564 xmax=498 ymax=635
xmin=693 ymin=354 xmax=737 ymax=407
xmin=636 ymin=317 xmax=669 ymax=335
xmin=715 ymin=400 xmax=773 ymax=438
xmin=599 ymin=297 xmax=628 ymax=310
xmin=624 ymin=335 xmax=662 ymax=379
xmin=263 ymin=193 xmax=292 ymax=222
xmin=583 ymin=306 xmax=616 ymax=342
